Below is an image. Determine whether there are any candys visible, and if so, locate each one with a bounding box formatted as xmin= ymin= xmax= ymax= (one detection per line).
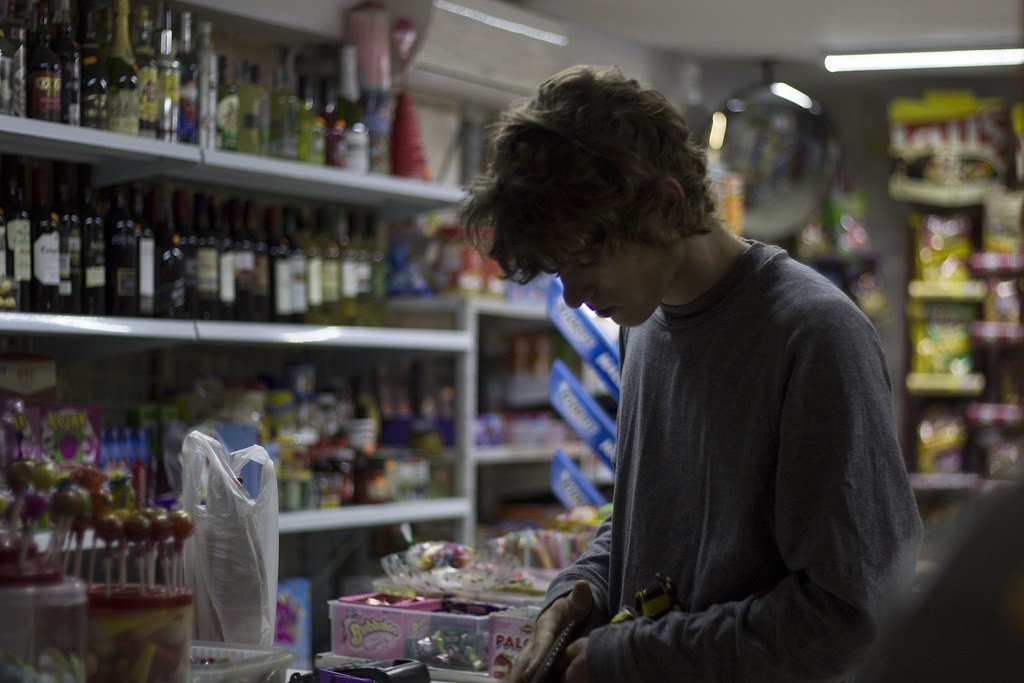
xmin=403 ymin=539 xmax=477 ymax=571
xmin=405 ymin=627 xmax=489 ymax=673
xmin=0 ymin=458 xmax=195 ymax=596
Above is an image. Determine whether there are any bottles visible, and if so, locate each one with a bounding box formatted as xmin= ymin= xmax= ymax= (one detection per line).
xmin=0 ymin=0 xmax=389 ymax=328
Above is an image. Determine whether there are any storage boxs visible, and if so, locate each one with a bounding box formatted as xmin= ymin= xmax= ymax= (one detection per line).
xmin=0 ymin=88 xmax=1024 ymax=683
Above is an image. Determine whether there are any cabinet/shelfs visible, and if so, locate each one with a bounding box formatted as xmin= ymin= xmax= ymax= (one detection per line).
xmin=387 ymin=294 xmax=589 ymax=459
xmin=0 ymin=113 xmax=480 ymax=560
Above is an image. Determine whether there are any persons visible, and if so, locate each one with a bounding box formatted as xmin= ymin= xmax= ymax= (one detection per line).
xmin=461 ymin=67 xmax=924 ymax=683
xmin=865 ymin=482 xmax=1024 ymax=683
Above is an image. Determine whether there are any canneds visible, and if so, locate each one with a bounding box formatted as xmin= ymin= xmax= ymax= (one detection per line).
xmin=318 ymin=447 xmax=393 ymax=505
